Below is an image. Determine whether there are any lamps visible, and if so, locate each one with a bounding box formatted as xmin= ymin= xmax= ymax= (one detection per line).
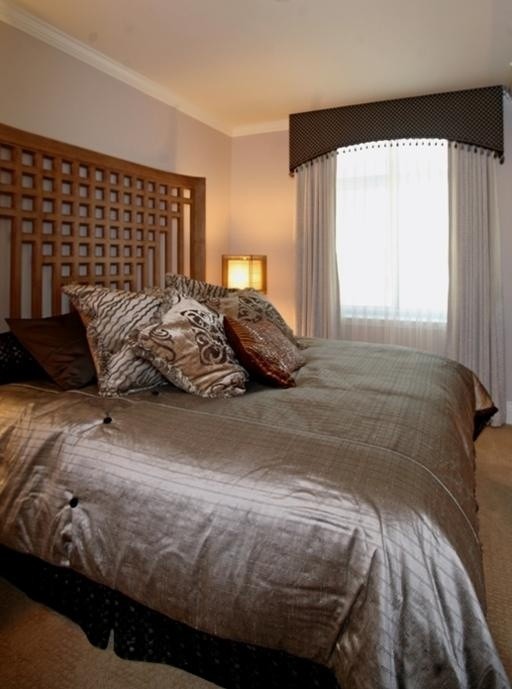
xmin=219 ymin=253 xmax=269 ymax=294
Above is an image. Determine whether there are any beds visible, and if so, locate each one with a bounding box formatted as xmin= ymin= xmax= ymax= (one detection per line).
xmin=0 ymin=122 xmax=505 ymax=685
